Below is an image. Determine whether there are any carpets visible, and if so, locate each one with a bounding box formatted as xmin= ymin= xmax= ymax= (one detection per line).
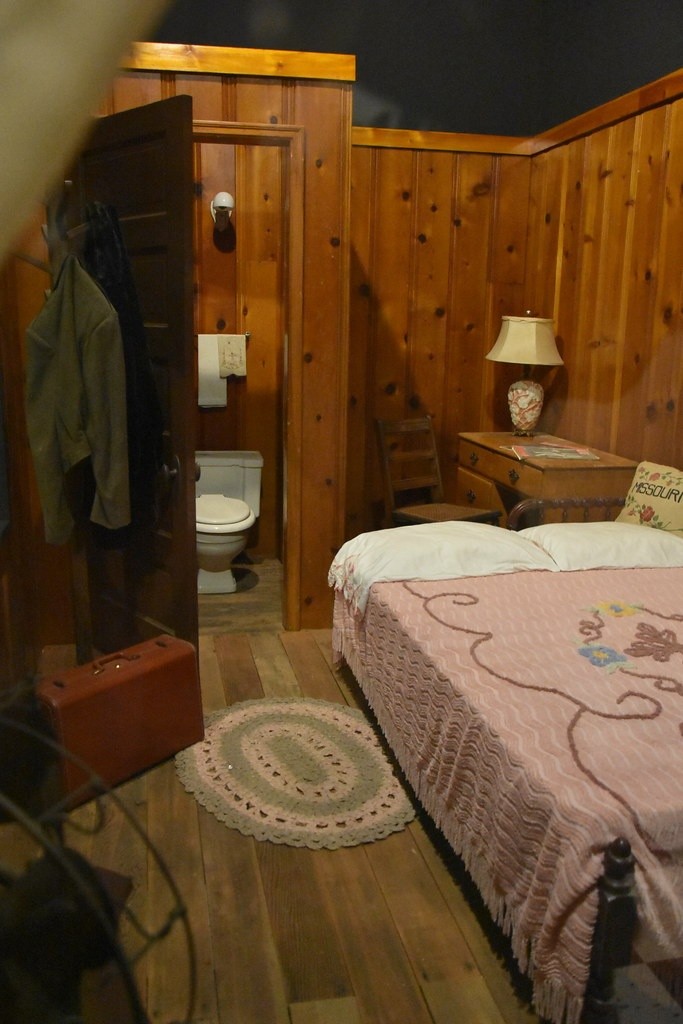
xmin=175 ymin=695 xmax=420 ymax=850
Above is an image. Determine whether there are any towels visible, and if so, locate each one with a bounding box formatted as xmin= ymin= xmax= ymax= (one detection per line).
xmin=197 ymin=334 xmax=225 ymax=407
xmin=217 ymin=334 xmax=246 ymax=378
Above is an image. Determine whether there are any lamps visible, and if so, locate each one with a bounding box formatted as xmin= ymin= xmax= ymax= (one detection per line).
xmin=483 ymin=309 xmax=566 ymax=436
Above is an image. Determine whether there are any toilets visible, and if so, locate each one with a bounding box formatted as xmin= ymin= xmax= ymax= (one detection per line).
xmin=195 ymin=451 xmax=264 ymax=594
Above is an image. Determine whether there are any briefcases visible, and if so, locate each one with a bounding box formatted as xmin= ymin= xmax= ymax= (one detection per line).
xmin=33 ymin=634 xmax=205 ymax=812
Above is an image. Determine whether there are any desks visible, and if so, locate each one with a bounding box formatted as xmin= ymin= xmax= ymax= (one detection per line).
xmin=458 ymin=425 xmax=640 ymax=523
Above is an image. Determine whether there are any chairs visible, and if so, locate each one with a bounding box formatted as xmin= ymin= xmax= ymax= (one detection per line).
xmin=371 ymin=413 xmax=502 ymax=527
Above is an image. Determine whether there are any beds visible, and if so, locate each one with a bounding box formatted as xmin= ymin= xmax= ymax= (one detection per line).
xmin=326 ymin=528 xmax=683 ymax=1024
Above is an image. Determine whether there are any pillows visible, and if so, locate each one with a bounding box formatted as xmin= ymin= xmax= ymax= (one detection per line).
xmin=329 ymin=520 xmax=562 ymax=624
xmin=616 ymin=461 xmax=683 ymax=537
xmin=519 ymin=521 xmax=683 ymax=572
